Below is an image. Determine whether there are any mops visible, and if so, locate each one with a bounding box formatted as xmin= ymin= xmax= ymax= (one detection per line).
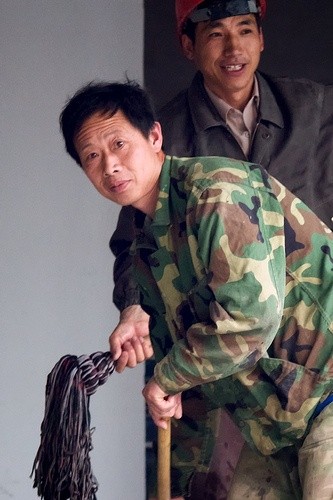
xmin=28 ymin=351 xmax=117 ymax=500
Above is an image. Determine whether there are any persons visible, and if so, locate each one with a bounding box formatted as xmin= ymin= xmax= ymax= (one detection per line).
xmin=55 ymin=74 xmax=333 ymax=500
xmin=107 ymin=0 xmax=333 ymax=500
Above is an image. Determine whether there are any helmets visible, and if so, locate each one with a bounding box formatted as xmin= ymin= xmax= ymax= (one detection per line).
xmin=174 ymin=0 xmax=266 ymax=56
